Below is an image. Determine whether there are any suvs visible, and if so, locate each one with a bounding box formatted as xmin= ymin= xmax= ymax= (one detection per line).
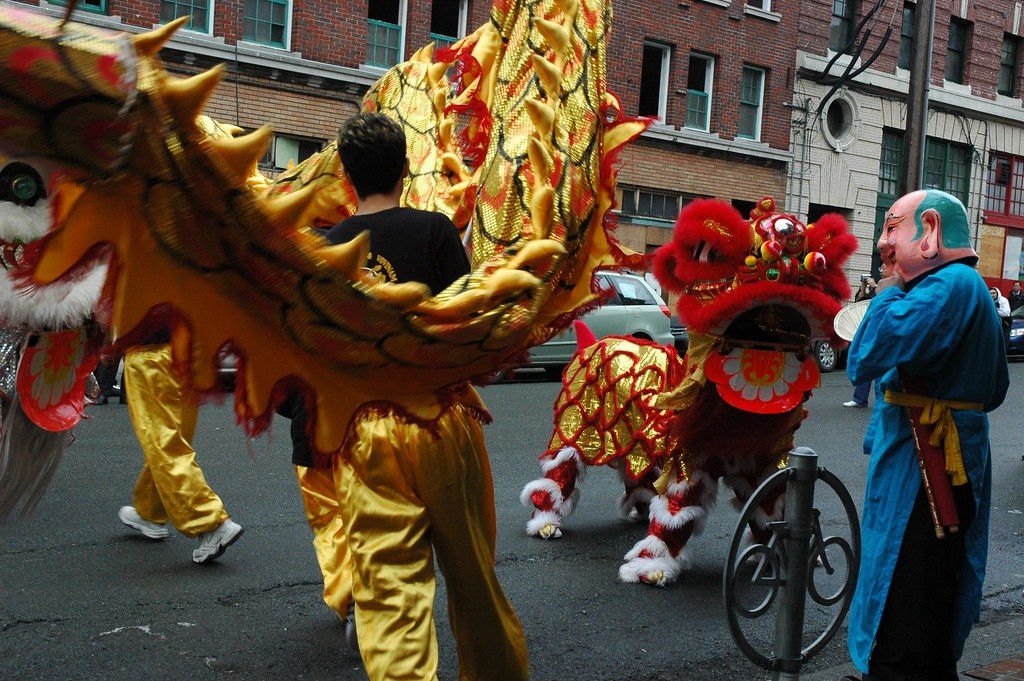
xmin=472 ymin=268 xmax=675 ymax=384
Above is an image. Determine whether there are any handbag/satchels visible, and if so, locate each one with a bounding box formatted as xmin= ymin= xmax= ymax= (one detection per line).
xmin=1002 ymin=317 xmax=1012 ymax=329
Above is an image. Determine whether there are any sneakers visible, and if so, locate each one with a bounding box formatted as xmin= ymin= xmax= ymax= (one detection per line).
xmin=192 ymin=516 xmax=244 ymax=563
xmin=118 ymin=506 xmax=169 ymax=538
xmin=843 ymin=401 xmax=867 ymax=408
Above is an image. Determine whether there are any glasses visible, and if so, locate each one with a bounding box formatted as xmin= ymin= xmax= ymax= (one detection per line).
xmin=878 ymin=267 xmax=886 ymax=272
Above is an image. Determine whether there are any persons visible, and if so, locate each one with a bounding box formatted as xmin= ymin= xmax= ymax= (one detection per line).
xmin=85 ymin=240 xmax=246 ymax=564
xmin=847 ymin=190 xmax=1011 ymax=681
xmin=843 ymin=262 xmax=1023 ymax=409
xmin=272 ymin=110 xmax=529 ymax=681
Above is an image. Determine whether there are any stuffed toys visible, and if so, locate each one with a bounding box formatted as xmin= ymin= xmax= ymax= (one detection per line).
xmin=519 ymin=195 xmax=860 ymax=589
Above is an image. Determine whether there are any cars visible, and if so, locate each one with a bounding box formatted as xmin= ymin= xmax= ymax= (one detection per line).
xmin=670 ymin=313 xmax=841 ymax=372
xmin=113 ymin=343 xmax=236 ymax=394
xmin=1007 ymin=305 xmax=1024 ymax=360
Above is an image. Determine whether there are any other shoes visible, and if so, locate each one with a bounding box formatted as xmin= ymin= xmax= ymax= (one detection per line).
xmin=344 ymin=602 xmax=360 ymax=650
xmin=97 ymin=398 xmax=108 ymax=404
xmin=120 ymin=399 xmax=127 ymax=404
